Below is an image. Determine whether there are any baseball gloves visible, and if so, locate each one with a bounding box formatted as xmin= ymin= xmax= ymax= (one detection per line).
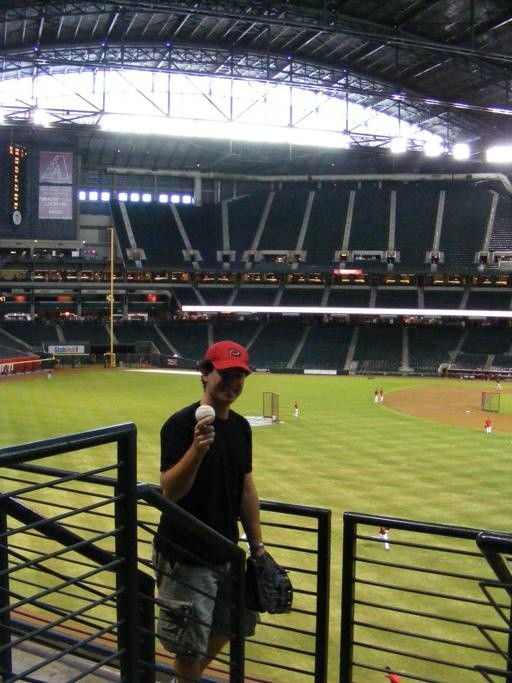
xmin=234 ymin=551 xmax=292 ymax=613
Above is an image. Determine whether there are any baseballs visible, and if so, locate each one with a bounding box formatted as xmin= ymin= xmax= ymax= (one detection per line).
xmin=195 ymin=404 xmax=215 ymax=423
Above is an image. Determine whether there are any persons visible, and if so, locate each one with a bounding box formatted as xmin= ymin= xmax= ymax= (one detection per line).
xmin=384 ymin=665 xmax=400 ymax=682
xmin=370 ymin=526 xmax=391 ymax=550
xmin=484 ymin=416 xmax=491 ymax=434
xmin=154 ymin=338 xmax=294 ymax=681
xmin=294 ymin=400 xmax=299 ymax=416
xmin=47 ymin=368 xmax=53 ymax=378
xmin=379 ymin=386 xmax=383 ymax=401
xmin=495 ymin=377 xmax=502 ymax=391
xmin=373 ymin=387 xmax=378 ymax=401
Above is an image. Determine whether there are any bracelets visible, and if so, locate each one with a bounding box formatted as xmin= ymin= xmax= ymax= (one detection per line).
xmin=249 ymin=541 xmax=264 ymax=551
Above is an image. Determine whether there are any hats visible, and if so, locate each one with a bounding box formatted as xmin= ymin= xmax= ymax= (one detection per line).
xmin=204 ymin=340 xmax=251 ymax=376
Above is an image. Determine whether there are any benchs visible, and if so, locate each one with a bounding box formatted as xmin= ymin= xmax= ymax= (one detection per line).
xmin=0 ymin=176 xmax=510 ymax=382
